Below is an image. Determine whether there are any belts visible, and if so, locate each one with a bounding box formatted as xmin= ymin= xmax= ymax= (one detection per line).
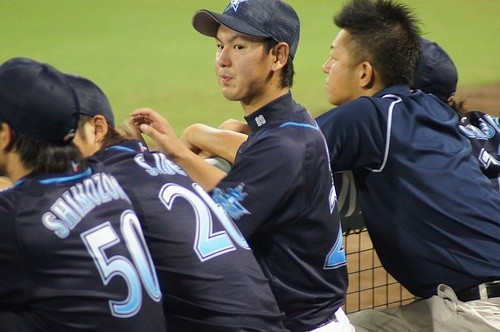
xmin=453 ymin=282 xmax=500 ymax=303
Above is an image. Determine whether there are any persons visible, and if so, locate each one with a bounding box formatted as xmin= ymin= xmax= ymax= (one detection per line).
xmin=410 ymin=37 xmax=500 ymax=192
xmin=179 ymin=0 xmax=500 ymax=332
xmin=0 ymin=72 xmax=286 ymax=332
xmin=0 ymin=57 xmax=164 ymax=332
xmin=113 ymin=0 xmax=355 ymax=332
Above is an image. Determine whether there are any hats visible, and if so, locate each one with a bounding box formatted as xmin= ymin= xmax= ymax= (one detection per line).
xmin=410 ymin=38 xmax=458 ymax=103
xmin=0 ymin=56 xmax=81 ymax=146
xmin=65 ymin=74 xmax=116 ymax=128
xmin=191 ymin=0 xmax=301 ymax=59
